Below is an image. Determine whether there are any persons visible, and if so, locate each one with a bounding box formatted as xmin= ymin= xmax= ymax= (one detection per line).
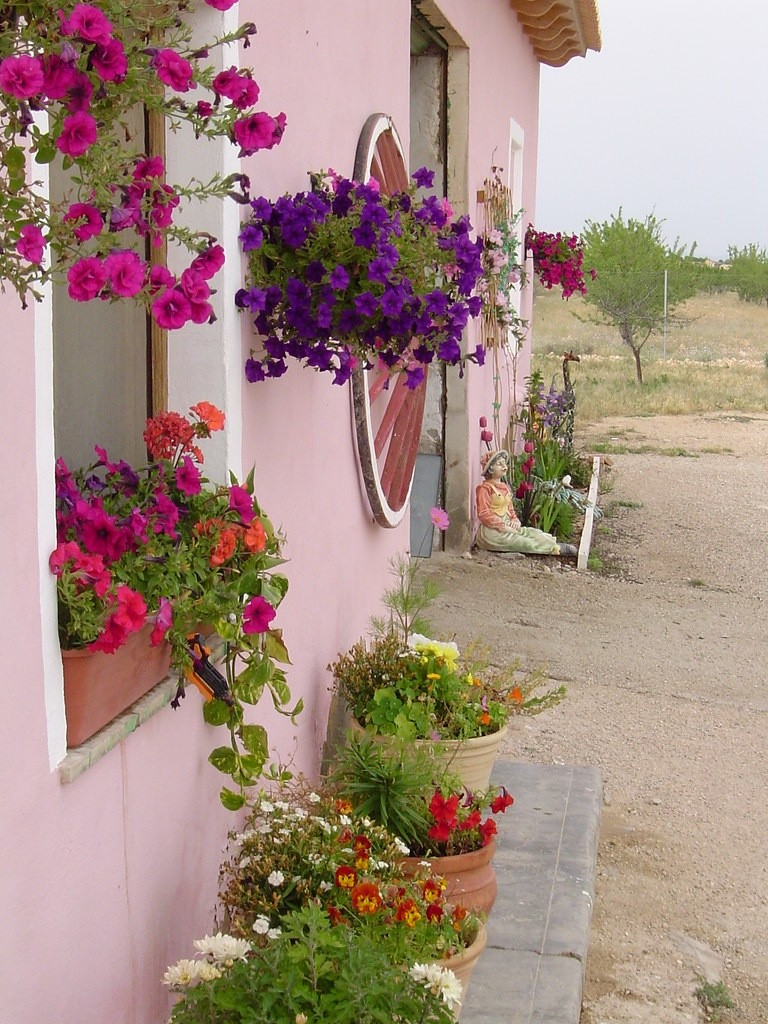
xmin=476 ymin=449 xmax=577 ymax=556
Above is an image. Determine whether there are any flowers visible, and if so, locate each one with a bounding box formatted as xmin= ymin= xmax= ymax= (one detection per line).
xmin=157 ymin=737 xmax=480 ymax=1024
xmin=326 ymin=505 xmax=567 ymax=741
xmin=479 ymin=206 xmax=540 ymax=347
xmin=346 ymin=712 xmax=506 ymax=804
xmin=523 ymin=222 xmax=599 ymax=301
xmin=320 ymin=738 xmax=515 ymax=858
xmin=1 ymin=0 xmax=289 ymax=332
xmin=239 ymin=167 xmax=493 ymax=390
xmin=50 ymin=399 xmax=306 ymax=815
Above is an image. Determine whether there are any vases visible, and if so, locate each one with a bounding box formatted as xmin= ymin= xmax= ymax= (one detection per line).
xmin=370 ymin=832 xmax=499 ymax=923
xmin=63 ymin=594 xmax=222 ymax=750
xmin=367 ymin=912 xmax=488 ymax=1023
